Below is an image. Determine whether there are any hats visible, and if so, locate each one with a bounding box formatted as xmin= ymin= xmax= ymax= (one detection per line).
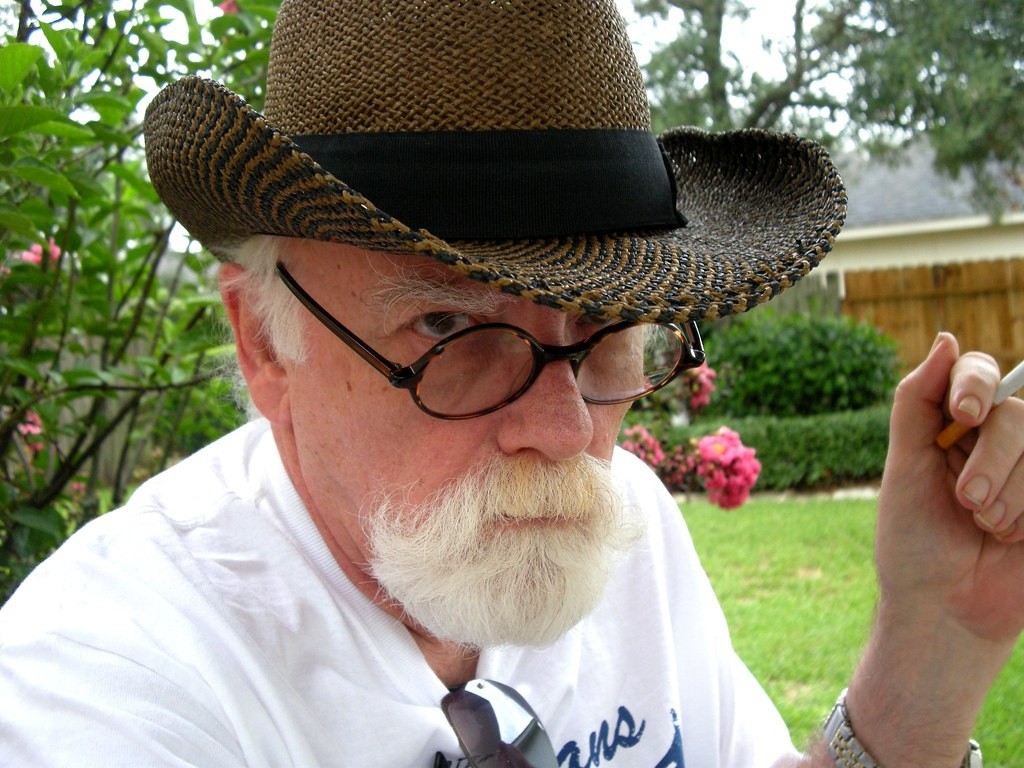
xmin=145 ymin=0 xmax=846 ymax=323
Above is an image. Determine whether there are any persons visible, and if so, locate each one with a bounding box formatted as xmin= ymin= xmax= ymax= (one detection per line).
xmin=1 ymin=1 xmax=1024 ymax=768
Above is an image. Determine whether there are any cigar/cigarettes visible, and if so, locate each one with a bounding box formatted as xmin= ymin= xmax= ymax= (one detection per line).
xmin=935 ymin=361 xmax=1024 ymax=449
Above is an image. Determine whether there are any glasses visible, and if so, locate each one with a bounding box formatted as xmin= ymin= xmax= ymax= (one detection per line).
xmin=434 ymin=679 xmax=557 ymax=768
xmin=271 ymin=260 xmax=706 ymax=420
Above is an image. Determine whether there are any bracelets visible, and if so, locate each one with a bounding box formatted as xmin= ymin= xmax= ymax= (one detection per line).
xmin=823 ymin=686 xmax=983 ymax=768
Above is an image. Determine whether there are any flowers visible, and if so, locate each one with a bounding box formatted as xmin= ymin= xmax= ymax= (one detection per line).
xmin=14 ymin=410 xmax=46 ymax=450
xmin=619 ymin=357 xmax=762 ymax=511
xmin=10 ymin=238 xmax=61 ymax=266
